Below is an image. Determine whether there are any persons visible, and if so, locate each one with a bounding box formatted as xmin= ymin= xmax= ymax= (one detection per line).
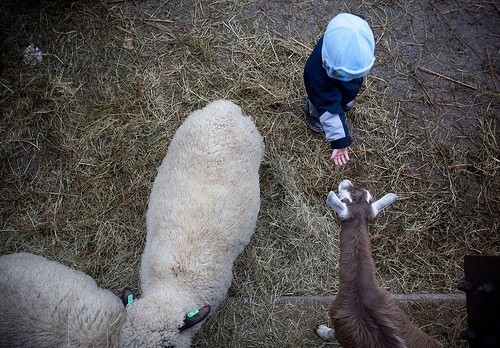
xmin=303 ymin=12 xmax=375 ymax=166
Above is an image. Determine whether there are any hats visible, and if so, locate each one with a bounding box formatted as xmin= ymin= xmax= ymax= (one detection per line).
xmin=322 ymin=13 xmax=376 ymax=82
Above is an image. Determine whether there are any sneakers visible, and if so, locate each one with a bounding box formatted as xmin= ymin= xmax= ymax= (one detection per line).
xmin=342 ymin=100 xmax=353 ymax=110
xmin=304 ymin=98 xmax=325 ymax=133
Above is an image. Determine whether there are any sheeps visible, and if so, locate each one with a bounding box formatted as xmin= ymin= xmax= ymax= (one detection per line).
xmin=0 ymin=99 xmax=265 ymax=348
xmin=314 ymin=178 xmax=446 ymax=348
